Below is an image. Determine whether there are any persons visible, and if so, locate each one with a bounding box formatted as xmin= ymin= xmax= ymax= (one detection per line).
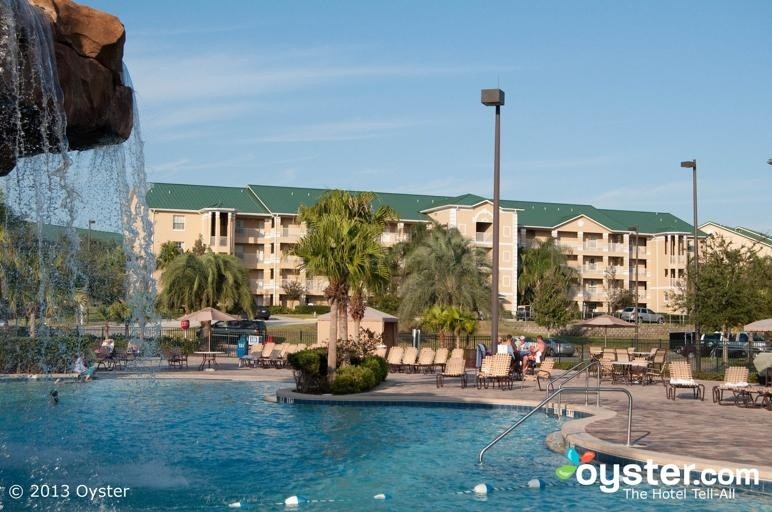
xmin=501 ymin=334 xmax=545 ymax=378
xmin=74 ymin=352 xmax=91 ymax=381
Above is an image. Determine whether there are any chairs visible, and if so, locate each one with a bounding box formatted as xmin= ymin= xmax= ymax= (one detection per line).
xmin=164 ymin=348 xmax=188 ymax=368
xmin=588 ymin=345 xmax=772 ymax=412
xmin=93 ymin=348 xmax=144 ymax=370
xmin=238 ymin=339 xmax=557 ymax=393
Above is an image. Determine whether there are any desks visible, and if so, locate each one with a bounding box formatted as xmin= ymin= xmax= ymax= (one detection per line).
xmin=193 ymin=351 xmax=226 ymax=370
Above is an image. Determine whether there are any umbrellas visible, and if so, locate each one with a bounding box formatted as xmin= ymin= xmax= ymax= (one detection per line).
xmin=573 ymin=314 xmax=635 ymax=356
xmin=177 ymin=306 xmax=240 ymax=369
xmin=743 ymin=318 xmax=772 ymax=332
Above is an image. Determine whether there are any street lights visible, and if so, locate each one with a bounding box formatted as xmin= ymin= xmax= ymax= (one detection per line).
xmin=86 ymin=219 xmax=99 ymax=252
xmin=679 ymin=158 xmax=703 ymax=372
xmin=480 ymin=87 xmax=505 ymax=357
xmin=626 ymin=221 xmax=641 ymax=353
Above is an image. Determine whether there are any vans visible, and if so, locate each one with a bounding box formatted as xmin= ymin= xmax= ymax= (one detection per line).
xmin=700 ymin=330 xmax=767 ymax=353
xmin=196 ymin=320 xmax=268 ymax=346
xmin=516 ymin=305 xmax=533 ymax=322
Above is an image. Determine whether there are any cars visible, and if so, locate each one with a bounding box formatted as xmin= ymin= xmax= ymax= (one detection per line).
xmin=543 ymin=338 xmax=576 ymax=358
xmin=674 ymin=339 xmax=760 ymax=360
xmin=239 ymin=305 xmax=271 ymax=320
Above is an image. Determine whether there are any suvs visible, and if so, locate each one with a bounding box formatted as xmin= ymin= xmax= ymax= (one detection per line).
xmin=620 ymin=306 xmax=667 ymax=324
